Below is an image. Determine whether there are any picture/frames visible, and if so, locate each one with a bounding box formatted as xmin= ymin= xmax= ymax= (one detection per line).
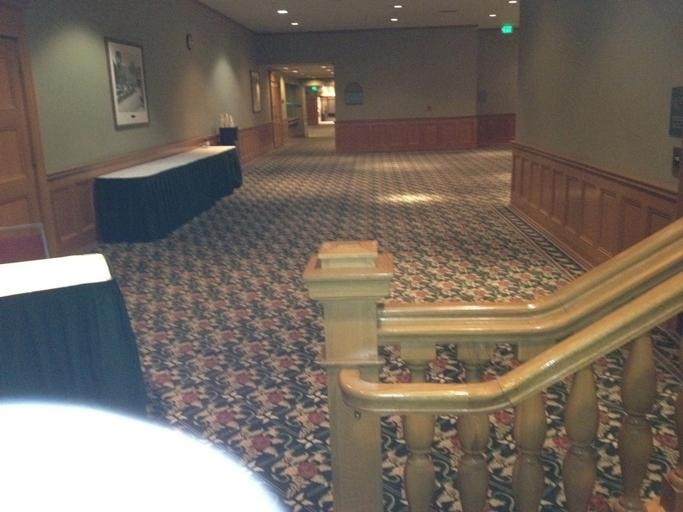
xmin=250 ymin=71 xmax=263 ymax=113
xmin=104 ymin=37 xmax=150 ymax=130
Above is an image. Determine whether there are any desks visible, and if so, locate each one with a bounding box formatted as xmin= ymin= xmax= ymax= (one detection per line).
xmin=92 ymin=144 xmax=242 ymax=243
xmin=0 ymin=253 xmax=145 ymax=423
